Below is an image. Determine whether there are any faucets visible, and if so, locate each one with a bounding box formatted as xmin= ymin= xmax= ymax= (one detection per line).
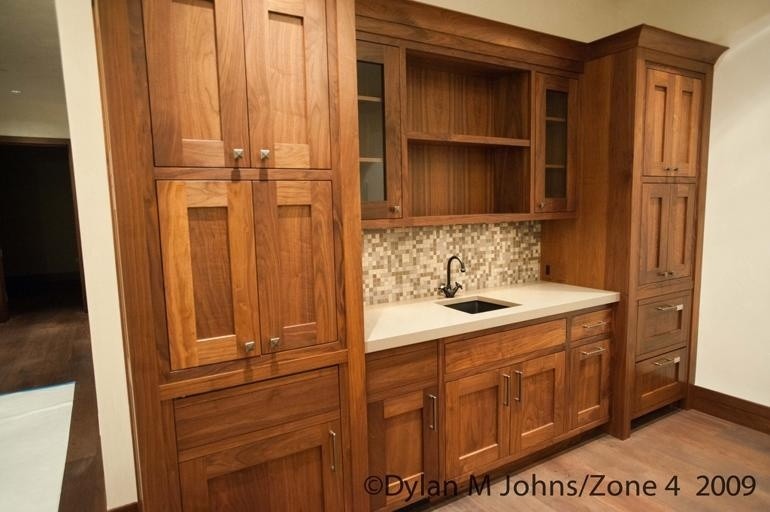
xmin=444 ymin=254 xmax=467 ymax=299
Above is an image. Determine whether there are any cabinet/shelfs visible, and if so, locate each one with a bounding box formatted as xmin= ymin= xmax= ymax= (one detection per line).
xmin=611 ymin=293 xmax=702 ymax=443
xmin=567 ymin=306 xmax=621 ymax=444
xmin=130 ymin=347 xmax=373 ymax=512
xmin=530 ymin=50 xmax=580 ymax=222
xmin=406 ymin=27 xmax=531 ymax=227
xmin=357 ymin=16 xmax=408 ymax=231
xmin=109 ymin=181 xmax=362 ymax=376
xmin=442 ymin=314 xmax=568 ymax=500
xmin=362 ymin=340 xmax=441 ymax=512
xmin=541 ymin=176 xmax=703 ymax=293
xmin=578 ymin=46 xmax=720 ymax=176
xmin=91 ymin=0 xmax=356 ymax=179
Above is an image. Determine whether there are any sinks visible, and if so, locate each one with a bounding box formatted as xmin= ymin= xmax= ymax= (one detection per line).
xmin=431 ymin=294 xmax=525 ymax=316
